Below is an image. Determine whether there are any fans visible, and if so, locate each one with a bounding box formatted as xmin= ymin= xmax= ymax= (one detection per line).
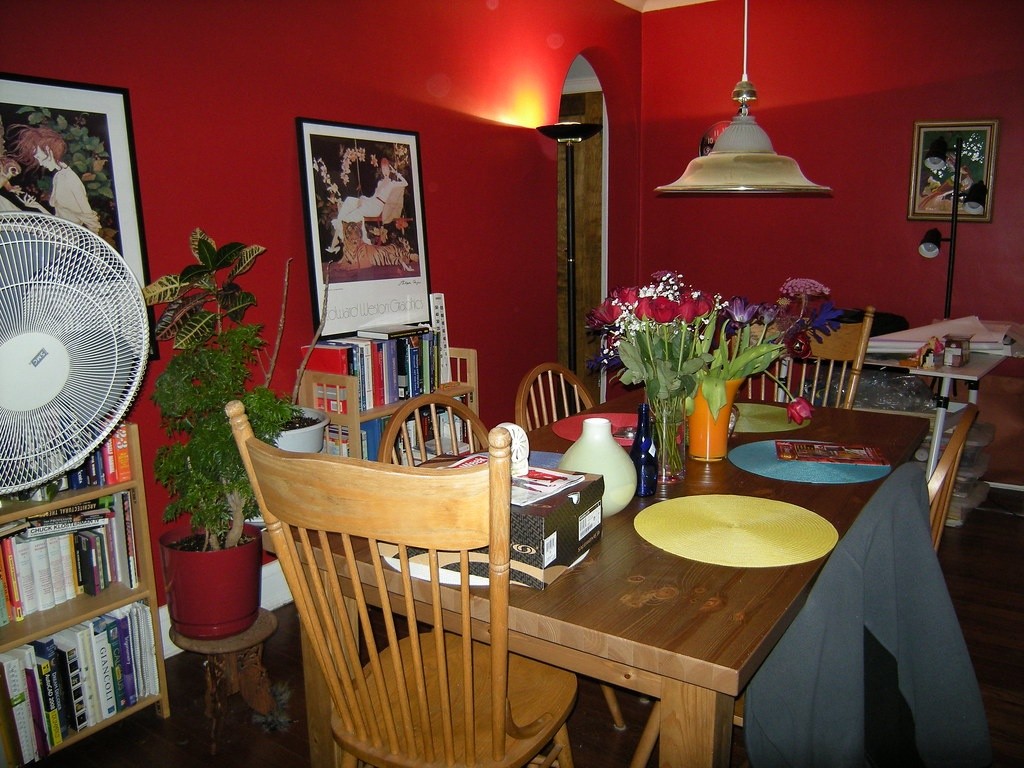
xmin=0 ymin=210 xmax=151 ymax=497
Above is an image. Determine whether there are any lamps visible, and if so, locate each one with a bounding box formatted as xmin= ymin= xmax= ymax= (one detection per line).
xmin=537 ymin=122 xmax=603 ymax=374
xmin=917 ymin=136 xmax=989 ymax=397
xmin=653 ymin=1 xmax=835 ymax=195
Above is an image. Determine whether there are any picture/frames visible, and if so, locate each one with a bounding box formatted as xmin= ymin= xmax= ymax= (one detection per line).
xmin=906 ymin=119 xmax=1000 ymax=223
xmin=295 ymin=116 xmax=434 ymax=339
xmin=0 ymin=71 xmax=161 ymax=364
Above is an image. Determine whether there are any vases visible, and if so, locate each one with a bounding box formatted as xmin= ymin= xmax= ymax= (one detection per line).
xmin=685 ymin=375 xmax=747 ymax=462
xmin=648 ymin=389 xmax=687 ymax=483
xmin=557 ymin=419 xmax=638 ymax=517
xmin=264 ymin=407 xmax=332 ymax=452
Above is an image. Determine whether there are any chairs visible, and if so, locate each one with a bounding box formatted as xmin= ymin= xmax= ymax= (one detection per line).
xmin=823 ymin=309 xmax=933 ymax=411
xmin=224 ymin=400 xmax=579 ymax=767
xmin=378 ymin=394 xmax=491 ymax=467
xmin=515 ymin=362 xmax=595 ymax=433
xmin=626 ymin=463 xmax=993 ymax=768
xmin=927 ymin=403 xmax=980 ymax=555
xmin=732 ymin=305 xmax=875 ymax=409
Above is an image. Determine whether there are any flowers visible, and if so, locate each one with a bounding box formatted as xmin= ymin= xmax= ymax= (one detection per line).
xmin=584 ymin=269 xmax=844 ymax=471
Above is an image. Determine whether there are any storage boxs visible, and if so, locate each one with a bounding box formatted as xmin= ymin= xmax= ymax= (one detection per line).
xmin=944 ymin=339 xmax=970 ymax=366
xmin=376 ymin=453 xmax=605 ymax=592
xmin=914 ymin=401 xmax=990 ymax=527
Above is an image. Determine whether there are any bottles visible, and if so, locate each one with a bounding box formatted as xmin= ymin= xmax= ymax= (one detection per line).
xmin=558 ymin=417 xmax=637 ymax=517
xmin=630 ymin=403 xmax=657 ymax=497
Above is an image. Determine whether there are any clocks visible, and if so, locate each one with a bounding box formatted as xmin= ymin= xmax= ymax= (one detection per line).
xmin=699 ymin=121 xmax=731 ymax=157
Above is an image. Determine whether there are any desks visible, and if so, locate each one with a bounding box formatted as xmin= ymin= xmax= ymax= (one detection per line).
xmin=793 ymin=351 xmax=1009 ymax=484
xmin=262 ymin=387 xmax=928 ymax=768
xmin=169 ymin=608 xmax=278 ymax=756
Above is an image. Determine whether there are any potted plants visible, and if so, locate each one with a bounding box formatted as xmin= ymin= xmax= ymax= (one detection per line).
xmin=141 ymin=226 xmax=304 ymax=640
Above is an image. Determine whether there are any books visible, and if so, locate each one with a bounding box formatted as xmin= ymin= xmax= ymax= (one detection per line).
xmin=301 ymin=324 xmax=446 ymax=412
xmin=0 ymin=490 xmax=141 ymax=626
xmin=0 ymin=421 xmax=134 ymax=497
xmin=319 ymin=396 xmax=469 ymax=466
xmin=0 ymin=599 xmax=160 ymax=765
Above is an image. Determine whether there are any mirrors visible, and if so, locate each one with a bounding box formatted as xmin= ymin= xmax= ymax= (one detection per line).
xmin=556 ymin=53 xmax=608 ymax=406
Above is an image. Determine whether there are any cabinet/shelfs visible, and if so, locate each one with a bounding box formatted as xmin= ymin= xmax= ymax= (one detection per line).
xmin=0 ymin=424 xmax=171 ymax=768
xmin=296 ymin=347 xmax=482 ymax=453
